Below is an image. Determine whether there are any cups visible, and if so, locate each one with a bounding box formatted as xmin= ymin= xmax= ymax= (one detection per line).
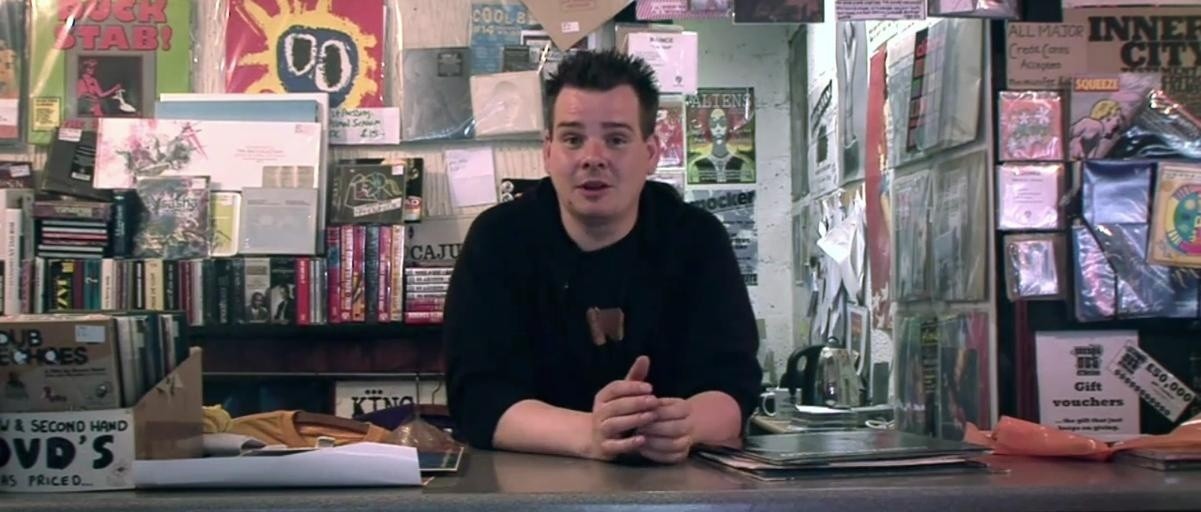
xmin=761 ymin=387 xmax=800 ymax=418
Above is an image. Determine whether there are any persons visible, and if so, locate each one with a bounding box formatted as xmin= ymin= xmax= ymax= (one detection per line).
xmin=687 ymin=95 xmax=755 ymax=183
xmin=77 ymin=58 xmax=136 ymax=112
xmin=444 ymin=47 xmax=763 ymax=465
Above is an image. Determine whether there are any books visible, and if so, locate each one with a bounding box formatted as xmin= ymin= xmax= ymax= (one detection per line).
xmin=94 ymin=92 xmax=455 ymax=326
xmin=0 ymin=91 xmax=211 ymax=411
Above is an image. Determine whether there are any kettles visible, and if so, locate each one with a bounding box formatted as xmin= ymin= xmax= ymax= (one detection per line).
xmin=784 ymin=335 xmax=865 ymax=409
